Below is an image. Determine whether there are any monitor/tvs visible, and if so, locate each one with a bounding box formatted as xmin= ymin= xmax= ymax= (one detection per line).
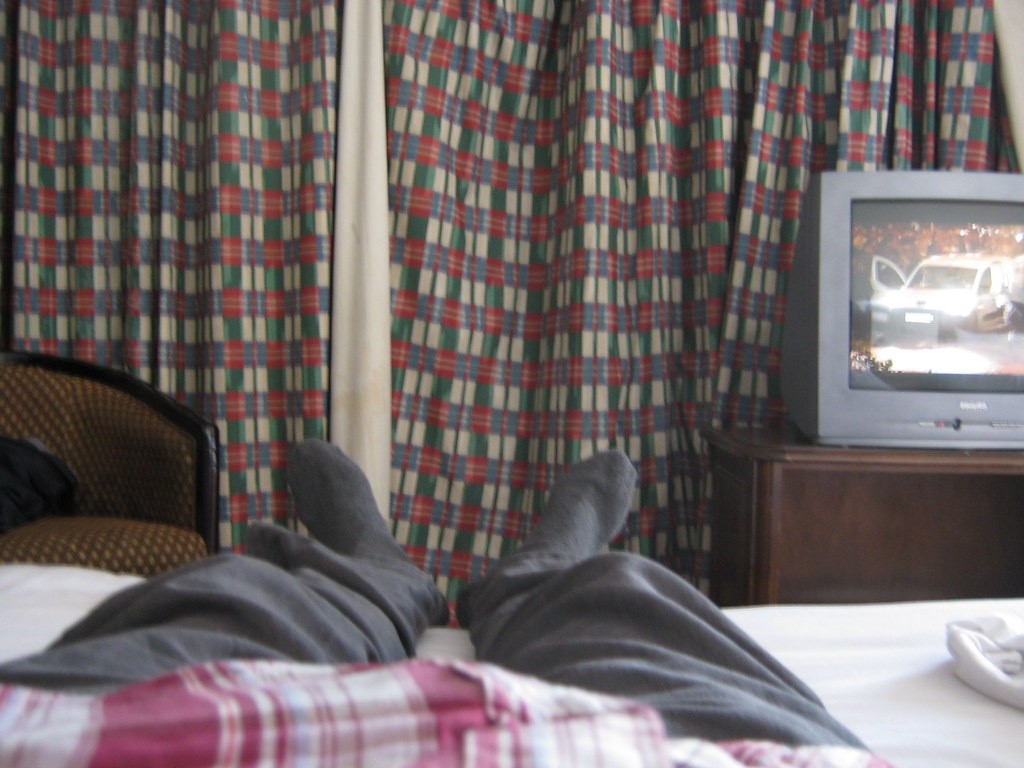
xmin=780 ymin=170 xmax=1024 ymax=452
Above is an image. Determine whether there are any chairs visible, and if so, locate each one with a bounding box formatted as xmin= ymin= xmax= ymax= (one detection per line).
xmin=0 ymin=348 xmax=219 ymax=582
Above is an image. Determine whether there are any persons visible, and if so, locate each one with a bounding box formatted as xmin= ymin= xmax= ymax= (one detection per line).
xmin=0 ymin=437 xmax=894 ymax=768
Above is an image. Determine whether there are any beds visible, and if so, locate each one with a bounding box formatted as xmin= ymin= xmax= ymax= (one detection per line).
xmin=0 ymin=563 xmax=1005 ymax=768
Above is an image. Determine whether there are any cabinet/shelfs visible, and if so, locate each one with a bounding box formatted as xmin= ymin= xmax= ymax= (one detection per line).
xmin=699 ymin=422 xmax=1023 ymax=606
